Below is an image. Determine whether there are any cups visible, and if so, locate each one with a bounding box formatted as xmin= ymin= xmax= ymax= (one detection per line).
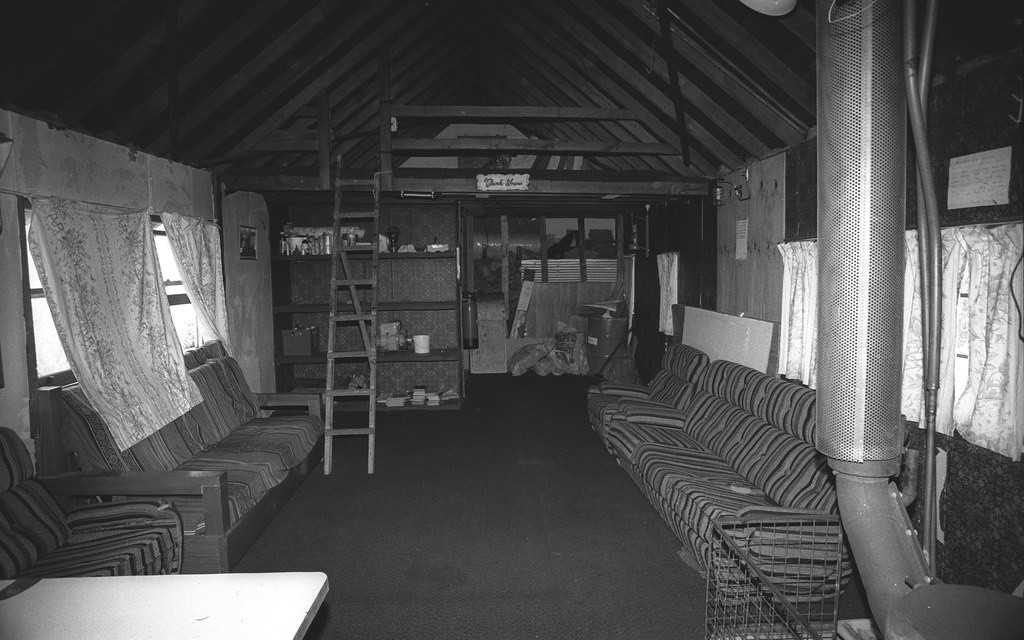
xmin=387 ymin=335 xmax=400 ymax=351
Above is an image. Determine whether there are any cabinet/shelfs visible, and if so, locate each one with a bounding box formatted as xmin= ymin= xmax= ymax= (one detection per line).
xmin=273 ymin=251 xmax=465 ymax=411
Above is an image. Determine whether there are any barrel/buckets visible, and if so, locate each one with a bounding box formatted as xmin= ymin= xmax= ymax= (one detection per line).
xmin=413 ymin=334 xmax=431 ymax=354
xmin=588 ymin=318 xmax=630 ymax=380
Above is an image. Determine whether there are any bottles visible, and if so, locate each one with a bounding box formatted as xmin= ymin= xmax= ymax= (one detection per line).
xmin=282 ymin=227 xmax=359 ymax=256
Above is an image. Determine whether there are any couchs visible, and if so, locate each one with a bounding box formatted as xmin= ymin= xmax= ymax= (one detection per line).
xmin=0 ymin=426 xmax=183 ymax=579
xmin=587 ymin=344 xmax=911 ymax=606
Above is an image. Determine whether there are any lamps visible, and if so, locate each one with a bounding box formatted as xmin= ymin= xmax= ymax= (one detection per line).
xmin=713 ymin=181 xmax=742 ymax=207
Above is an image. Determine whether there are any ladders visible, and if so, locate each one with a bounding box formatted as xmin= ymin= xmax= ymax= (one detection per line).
xmin=323 ymin=172 xmax=381 ymax=477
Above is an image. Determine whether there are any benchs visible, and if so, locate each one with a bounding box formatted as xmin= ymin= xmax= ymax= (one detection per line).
xmin=39 ymin=340 xmax=325 ymax=574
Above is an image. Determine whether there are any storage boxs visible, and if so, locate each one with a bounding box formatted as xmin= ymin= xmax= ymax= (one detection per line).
xmin=381 ymin=322 xmax=401 ymax=351
xmin=282 ymin=326 xmax=318 ymax=356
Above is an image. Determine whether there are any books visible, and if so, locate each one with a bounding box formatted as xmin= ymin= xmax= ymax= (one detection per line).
xmin=377 ymin=384 xmax=444 ymax=408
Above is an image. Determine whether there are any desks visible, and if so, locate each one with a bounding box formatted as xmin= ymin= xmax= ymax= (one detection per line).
xmin=1 ymin=572 xmax=329 ymax=640
